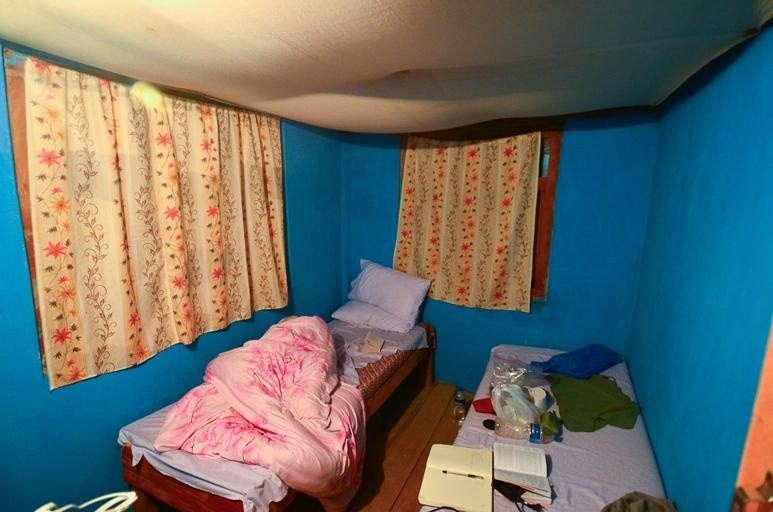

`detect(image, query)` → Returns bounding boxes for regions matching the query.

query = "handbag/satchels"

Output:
[544,373,640,432]
[531,344,623,380]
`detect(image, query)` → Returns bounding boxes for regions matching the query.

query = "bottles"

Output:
[494,417,563,443]
[452,384,465,427]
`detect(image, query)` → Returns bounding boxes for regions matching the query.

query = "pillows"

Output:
[331,300,418,334]
[346,259,433,323]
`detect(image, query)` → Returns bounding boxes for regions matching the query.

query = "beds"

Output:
[117,323,437,512]
[421,343,671,512]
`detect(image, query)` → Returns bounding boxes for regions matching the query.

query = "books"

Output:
[363,331,386,352]
[418,442,553,512]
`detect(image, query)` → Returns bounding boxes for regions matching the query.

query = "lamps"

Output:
[129,81,161,107]
